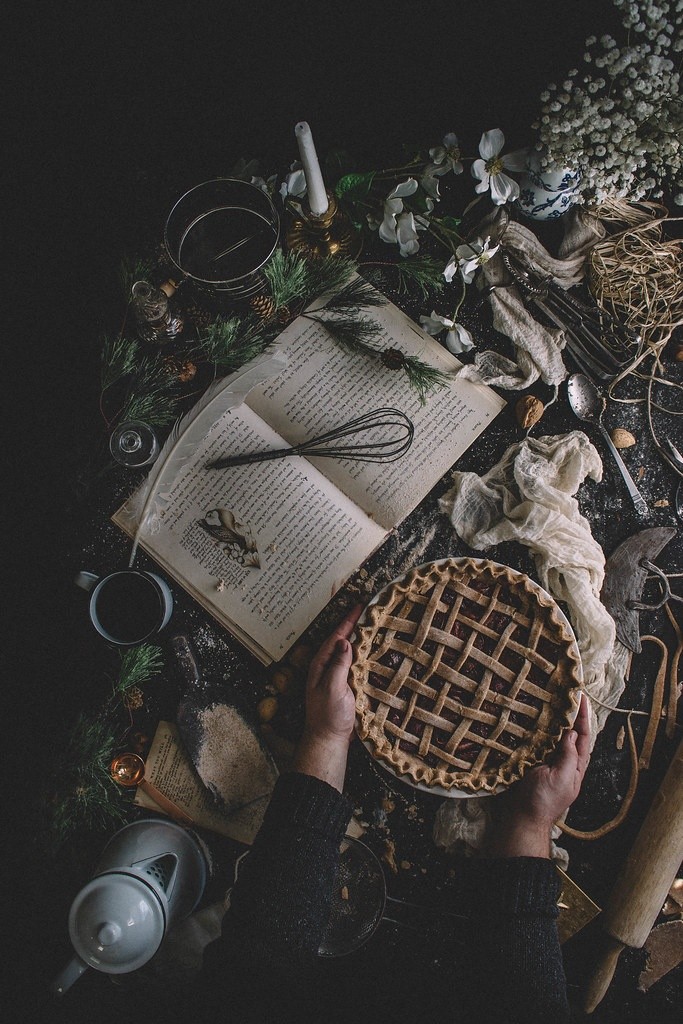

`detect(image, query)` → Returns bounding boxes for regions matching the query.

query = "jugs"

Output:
[43,819,206,995]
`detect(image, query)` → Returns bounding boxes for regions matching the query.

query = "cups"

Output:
[163,179,281,303]
[72,569,173,645]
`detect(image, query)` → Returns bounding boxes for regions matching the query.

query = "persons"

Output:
[186,603,593,1024]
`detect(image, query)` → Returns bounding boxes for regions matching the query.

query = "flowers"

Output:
[229,129,529,352]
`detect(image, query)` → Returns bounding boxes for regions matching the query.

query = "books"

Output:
[108,268,509,666]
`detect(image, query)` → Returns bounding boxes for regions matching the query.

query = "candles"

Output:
[295,121,329,214]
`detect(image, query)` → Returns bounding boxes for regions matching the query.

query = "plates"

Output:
[347,557,584,799]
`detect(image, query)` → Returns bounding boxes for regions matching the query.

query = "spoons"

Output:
[567,374,648,515]
[111,753,193,829]
